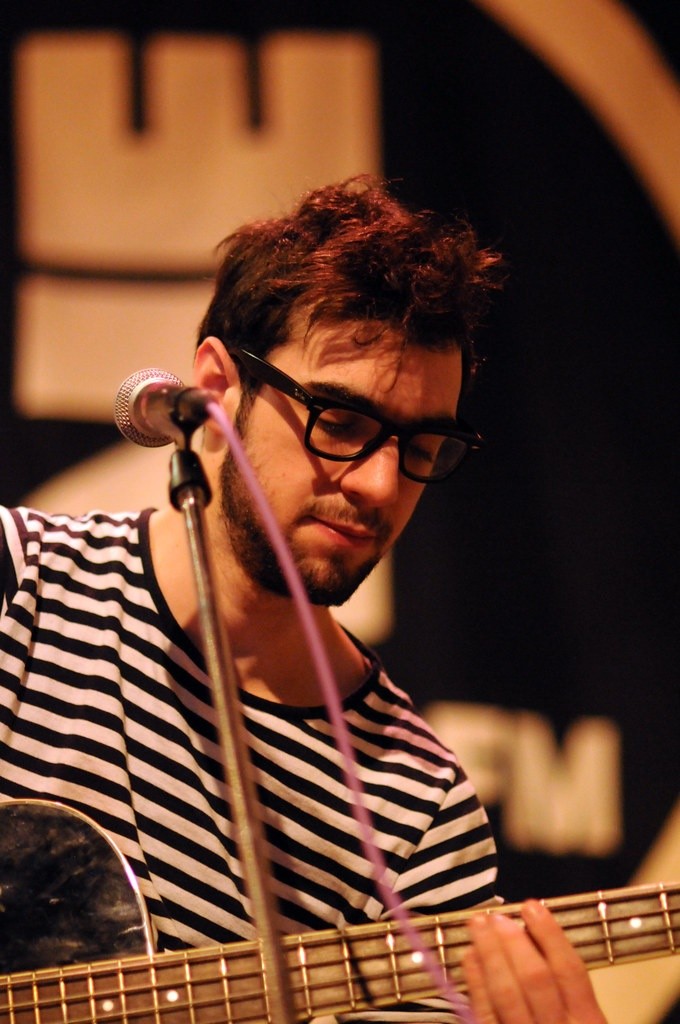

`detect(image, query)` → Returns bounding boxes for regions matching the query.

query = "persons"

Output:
[0,175,606,1023]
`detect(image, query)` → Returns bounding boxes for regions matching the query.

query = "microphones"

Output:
[114,368,220,448]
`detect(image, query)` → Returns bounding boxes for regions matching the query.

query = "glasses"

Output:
[228,345,489,485]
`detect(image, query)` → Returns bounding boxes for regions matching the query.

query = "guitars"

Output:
[0,793,679,1024]
